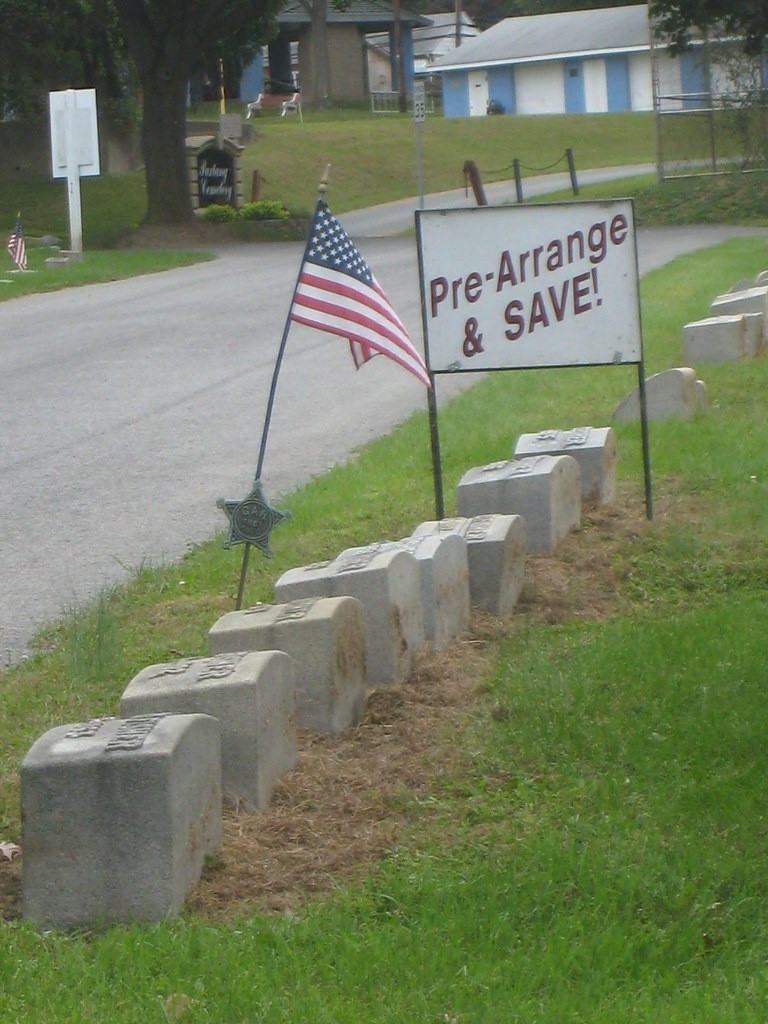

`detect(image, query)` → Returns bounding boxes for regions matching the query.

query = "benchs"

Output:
[246,92,302,120]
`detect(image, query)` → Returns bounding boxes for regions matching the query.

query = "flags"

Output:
[7,221,27,273]
[288,199,432,389]
